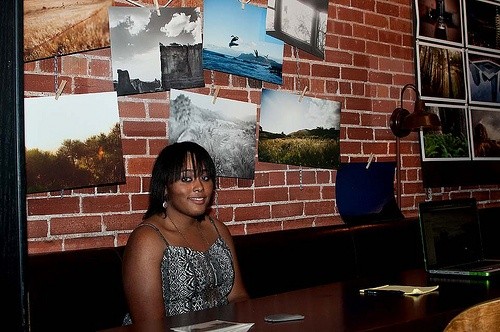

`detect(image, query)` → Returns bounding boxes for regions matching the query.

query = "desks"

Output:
[106,269,500,332]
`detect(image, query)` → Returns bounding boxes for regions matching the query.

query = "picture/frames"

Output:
[412,0,500,162]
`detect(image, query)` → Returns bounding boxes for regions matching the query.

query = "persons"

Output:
[122,141,249,326]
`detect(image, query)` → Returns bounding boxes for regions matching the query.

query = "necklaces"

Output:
[165,210,210,264]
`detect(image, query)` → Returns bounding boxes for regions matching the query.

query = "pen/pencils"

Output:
[364,289,406,295]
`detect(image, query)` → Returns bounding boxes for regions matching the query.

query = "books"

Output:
[359,285,439,294]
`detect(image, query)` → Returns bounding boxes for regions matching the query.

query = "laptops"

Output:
[417,197,499,280]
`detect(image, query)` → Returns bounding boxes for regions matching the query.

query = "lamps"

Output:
[389,83,442,138]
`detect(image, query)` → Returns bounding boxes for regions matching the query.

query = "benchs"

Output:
[27,206,500,332]
